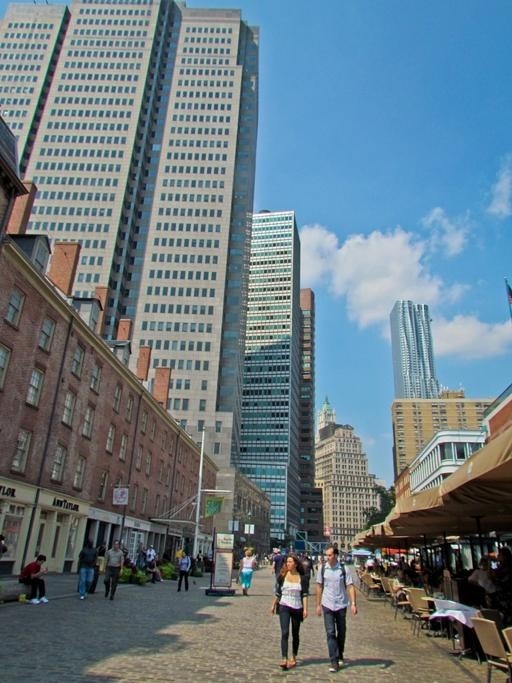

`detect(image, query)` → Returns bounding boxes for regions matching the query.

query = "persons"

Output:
[103,540,124,600]
[139,542,204,591]
[77,538,97,599]
[272,555,308,668]
[236,550,257,595]
[1,534,7,556]
[268,547,314,594]
[315,545,357,672]
[365,548,512,623]
[20,554,48,605]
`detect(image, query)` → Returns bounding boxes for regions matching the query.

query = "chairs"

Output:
[355,568,512,683]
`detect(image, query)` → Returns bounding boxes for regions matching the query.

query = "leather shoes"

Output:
[279,661,296,669]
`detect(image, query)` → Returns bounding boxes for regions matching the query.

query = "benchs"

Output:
[147,573,154,580]
[0,582,32,602]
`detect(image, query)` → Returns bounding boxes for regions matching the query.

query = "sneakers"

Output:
[329,663,339,673]
[80,593,88,600]
[29,599,40,605]
[39,597,48,603]
